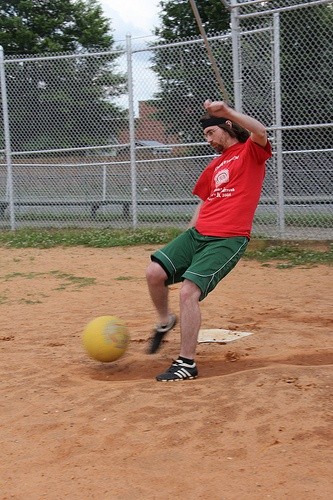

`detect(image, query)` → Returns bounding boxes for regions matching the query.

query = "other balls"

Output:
[82,315,129,364]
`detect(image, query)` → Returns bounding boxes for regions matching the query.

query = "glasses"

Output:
[203,127,219,140]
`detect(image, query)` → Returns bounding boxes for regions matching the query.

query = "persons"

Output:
[143,97,273,381]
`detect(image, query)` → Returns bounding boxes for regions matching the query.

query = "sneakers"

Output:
[149,317,177,354]
[156,361,198,382]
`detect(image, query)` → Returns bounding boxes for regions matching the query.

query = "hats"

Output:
[198,113,228,130]
